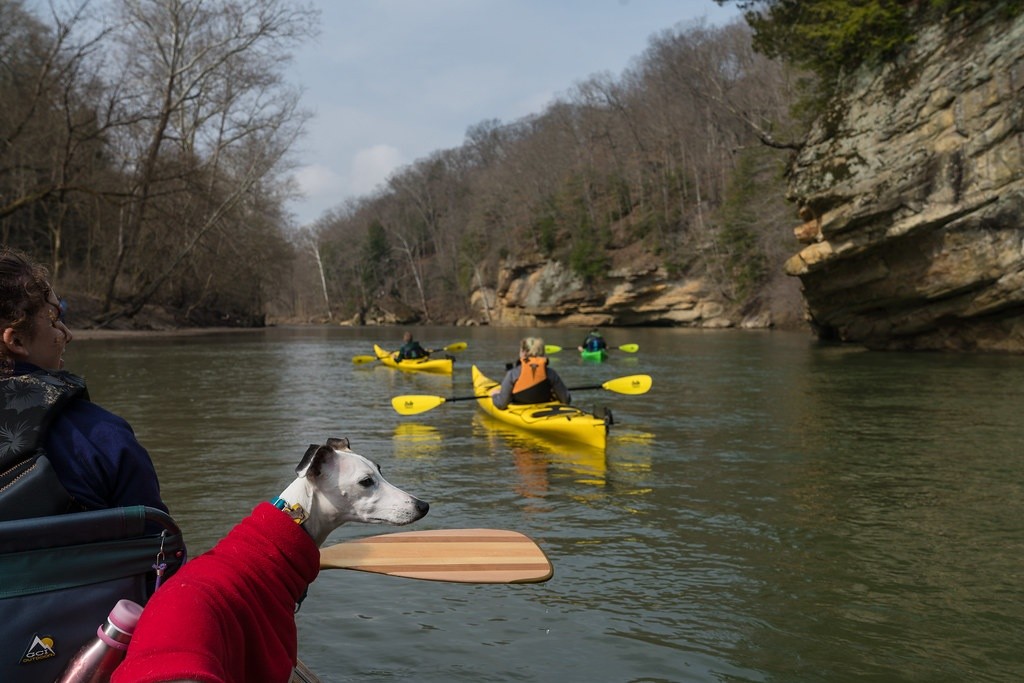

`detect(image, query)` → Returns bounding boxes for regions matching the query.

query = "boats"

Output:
[579,346,608,366]
[372,343,453,375]
[470,363,614,449]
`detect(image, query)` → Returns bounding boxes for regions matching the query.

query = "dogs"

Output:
[110,437,430,683]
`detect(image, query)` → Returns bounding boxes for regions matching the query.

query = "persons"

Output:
[392,331,431,363]
[1,249,172,524]
[490,336,572,410]
[577,328,609,352]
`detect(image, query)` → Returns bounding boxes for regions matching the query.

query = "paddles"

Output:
[391,374,653,417]
[318,526,555,584]
[544,343,639,355]
[351,342,468,367]
